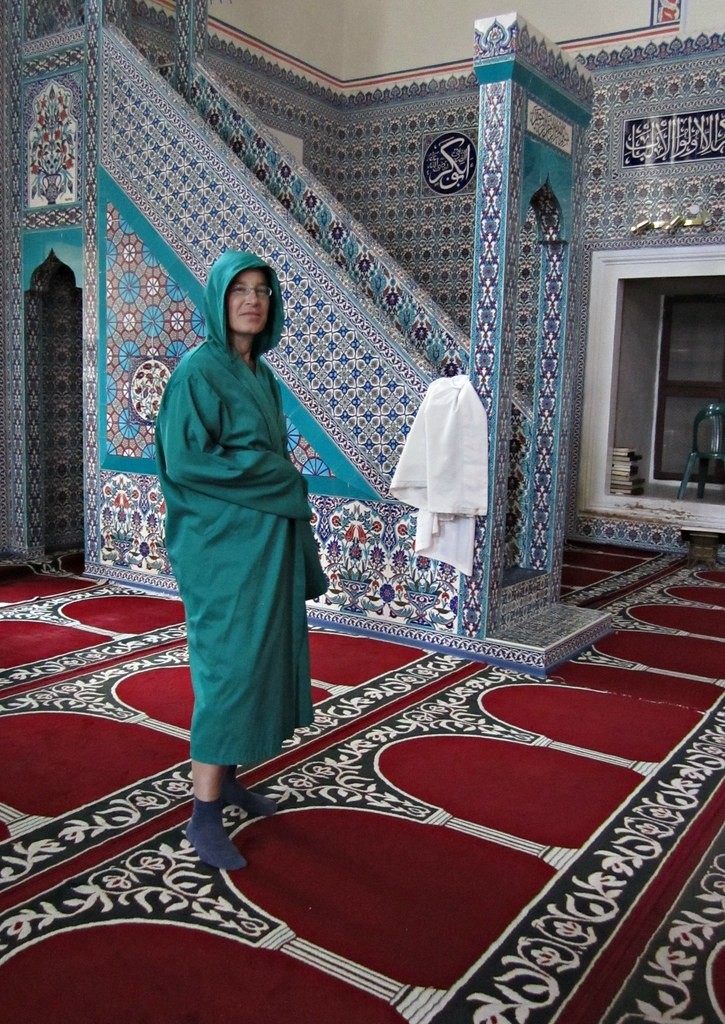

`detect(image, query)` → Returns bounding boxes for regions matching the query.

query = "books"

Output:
[609,446,646,497]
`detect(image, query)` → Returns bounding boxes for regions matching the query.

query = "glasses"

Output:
[230,282,273,299]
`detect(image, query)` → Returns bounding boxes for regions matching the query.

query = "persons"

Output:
[152,246,330,873]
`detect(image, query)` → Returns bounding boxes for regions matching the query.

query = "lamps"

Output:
[630,219,652,238]
[700,207,712,228]
[661,215,683,236]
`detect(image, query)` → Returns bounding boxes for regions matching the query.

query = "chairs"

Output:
[676,402,725,500]
